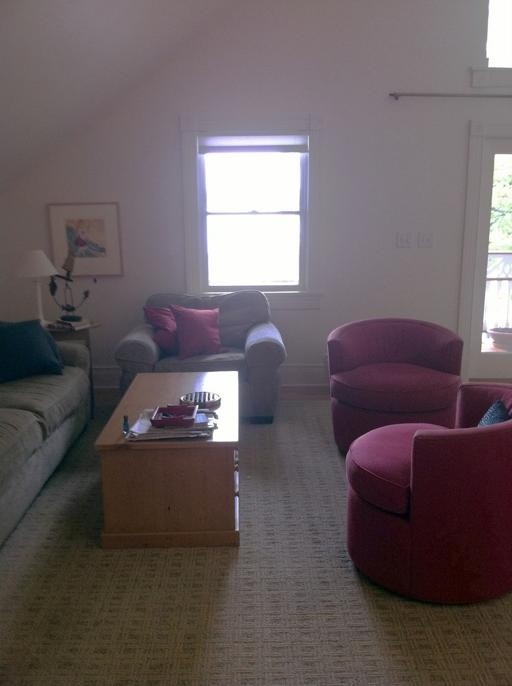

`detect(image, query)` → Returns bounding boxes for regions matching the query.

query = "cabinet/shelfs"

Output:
[95,370,243,549]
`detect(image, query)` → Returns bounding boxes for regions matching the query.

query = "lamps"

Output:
[10,249,60,327]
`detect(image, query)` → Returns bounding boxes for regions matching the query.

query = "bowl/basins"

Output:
[489,327,512,343]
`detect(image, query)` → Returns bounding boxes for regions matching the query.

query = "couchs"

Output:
[114,289,288,424]
[0,340,92,549]
[327,316,464,453]
[346,381,511,605]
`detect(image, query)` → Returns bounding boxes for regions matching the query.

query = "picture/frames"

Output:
[46,201,125,277]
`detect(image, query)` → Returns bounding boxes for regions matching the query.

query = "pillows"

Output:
[144,306,177,354]
[1,319,64,382]
[477,399,511,426]
[170,303,229,360]
[61,351,77,367]
